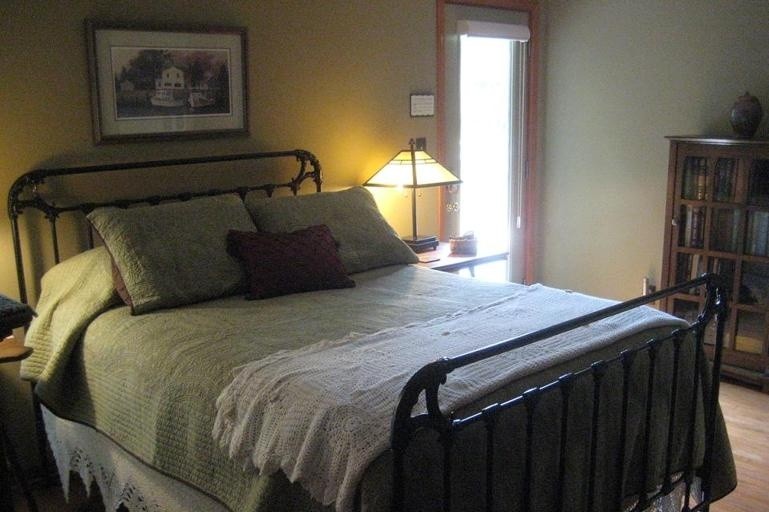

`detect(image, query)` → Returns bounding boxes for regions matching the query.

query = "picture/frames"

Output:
[82,15,249,137]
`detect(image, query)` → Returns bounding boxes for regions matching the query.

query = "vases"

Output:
[730,91,763,139]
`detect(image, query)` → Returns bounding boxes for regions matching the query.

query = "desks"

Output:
[410,239,510,277]
[0,337,38,512]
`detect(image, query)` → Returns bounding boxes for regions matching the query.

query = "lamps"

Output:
[363,139,462,250]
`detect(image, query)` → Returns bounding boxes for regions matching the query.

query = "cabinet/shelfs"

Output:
[659,134,769,394]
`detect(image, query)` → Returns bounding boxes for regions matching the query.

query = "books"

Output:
[674,153,769,310]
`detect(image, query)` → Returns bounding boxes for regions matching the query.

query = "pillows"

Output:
[88,189,259,318]
[246,184,420,273]
[225,221,357,299]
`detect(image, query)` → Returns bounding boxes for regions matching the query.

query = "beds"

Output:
[7,145,736,512]
